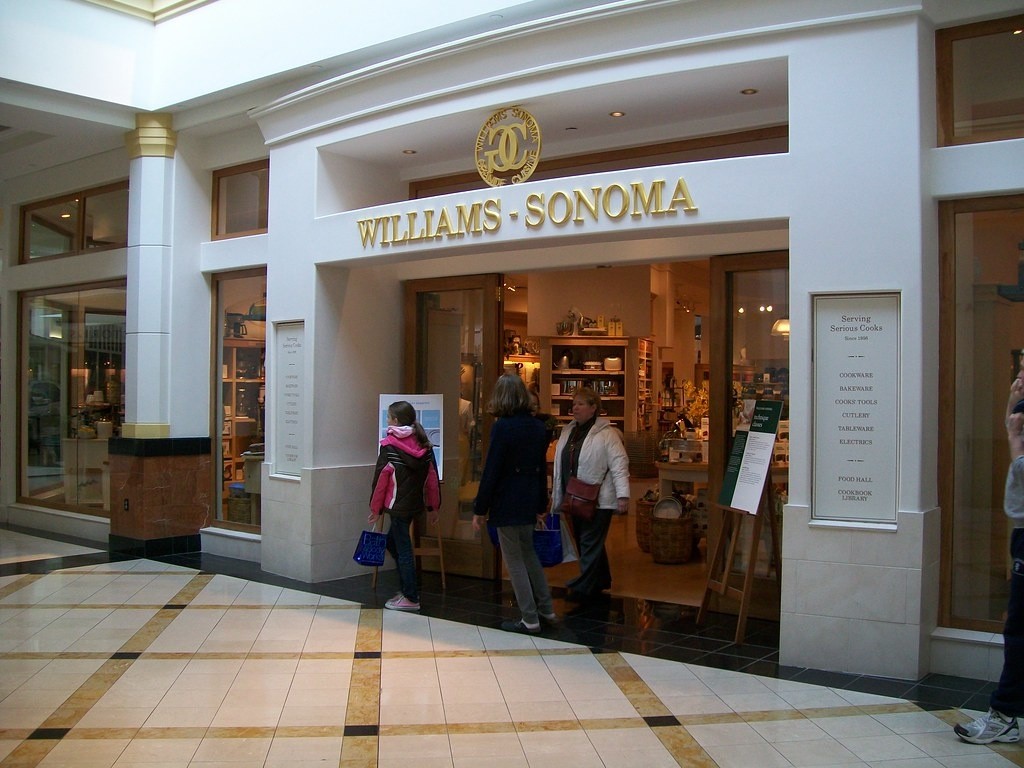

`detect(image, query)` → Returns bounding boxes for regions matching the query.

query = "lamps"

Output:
[770,316,790,337]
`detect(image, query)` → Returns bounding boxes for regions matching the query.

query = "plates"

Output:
[652,496,683,519]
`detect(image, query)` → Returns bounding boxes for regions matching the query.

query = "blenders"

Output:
[225,312,247,339]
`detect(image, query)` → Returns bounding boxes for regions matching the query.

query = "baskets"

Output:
[641,508,693,564]
[634,499,658,553]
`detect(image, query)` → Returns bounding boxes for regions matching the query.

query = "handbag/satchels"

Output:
[533,519,563,567]
[559,478,601,519]
[352,530,388,566]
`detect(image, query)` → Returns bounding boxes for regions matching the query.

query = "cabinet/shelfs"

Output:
[694,363,756,393]
[222,337,266,501]
[539,335,655,464]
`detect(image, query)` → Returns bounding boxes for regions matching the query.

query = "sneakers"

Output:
[536,610,558,624]
[565,576,612,600]
[954,707,1020,743]
[501,617,540,632]
[385,593,420,609]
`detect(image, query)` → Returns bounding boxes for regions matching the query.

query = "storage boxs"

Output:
[551,383,561,396]
[222,364,228,379]
[228,482,251,498]
[615,322,623,336]
[224,405,231,417]
[222,440,230,457]
[775,442,786,463]
[702,442,708,463]
[583,360,602,372]
[552,404,561,416]
[700,417,710,441]
[235,469,243,480]
[785,443,789,463]
[598,315,605,328]
[223,460,232,481]
[223,421,232,436]
[776,420,789,441]
[608,322,615,336]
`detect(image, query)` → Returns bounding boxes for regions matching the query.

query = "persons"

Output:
[369,401,442,611]
[954,348,1023,745]
[550,386,630,598]
[473,373,557,636]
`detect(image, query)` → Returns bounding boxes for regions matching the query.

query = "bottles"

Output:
[237,389,248,415]
[97,417,112,438]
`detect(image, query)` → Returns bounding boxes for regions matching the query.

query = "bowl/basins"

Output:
[86,395,96,402]
[77,429,95,439]
[94,391,104,401]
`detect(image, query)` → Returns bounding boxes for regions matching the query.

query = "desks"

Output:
[653,460,789,501]
[60,438,110,511]
[243,456,265,525]
